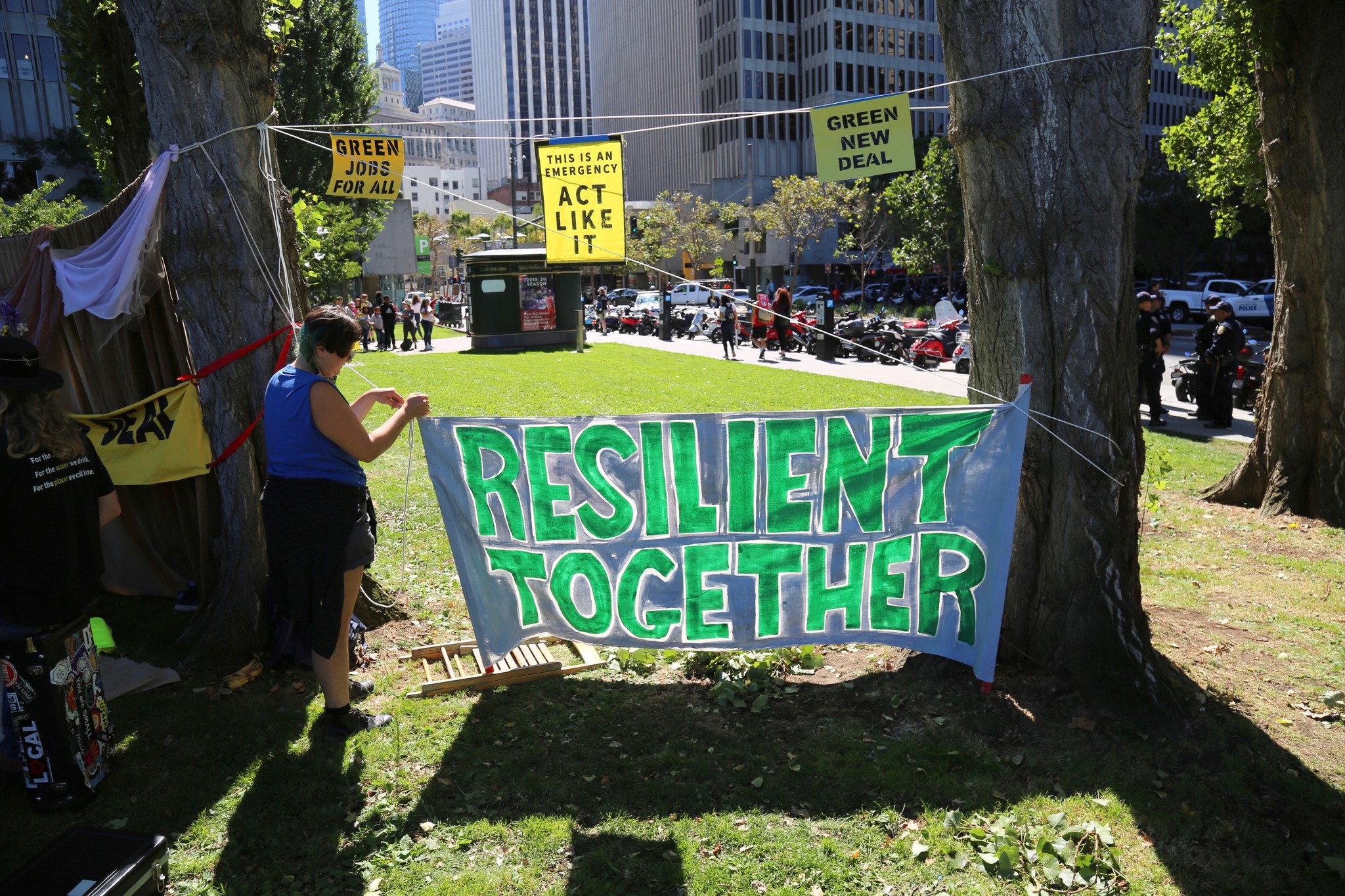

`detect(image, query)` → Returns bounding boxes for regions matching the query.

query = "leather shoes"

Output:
[1149,405,1225,430]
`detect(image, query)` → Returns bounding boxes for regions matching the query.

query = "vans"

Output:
[670,279,736,309]
[1184,271,1226,287]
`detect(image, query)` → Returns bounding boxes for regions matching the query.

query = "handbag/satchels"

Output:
[593,294,603,314]
[778,312,790,325]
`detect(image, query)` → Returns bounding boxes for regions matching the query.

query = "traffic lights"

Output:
[877,250,882,262]
[453,278,457,284]
[733,254,737,265]
[864,247,868,258]
[456,248,465,264]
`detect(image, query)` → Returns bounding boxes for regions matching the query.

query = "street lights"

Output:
[507,130,559,248]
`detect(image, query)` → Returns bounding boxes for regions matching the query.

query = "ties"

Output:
[1209,301,1232,312]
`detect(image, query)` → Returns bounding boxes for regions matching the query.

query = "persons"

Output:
[750,290,770,359]
[666,282,672,291]
[650,285,656,291]
[334,290,460,351]
[674,282,679,288]
[714,294,739,358]
[521,274,556,330]
[771,287,792,359]
[714,276,967,307]
[261,305,430,738]
[596,287,611,336]
[1,335,122,649]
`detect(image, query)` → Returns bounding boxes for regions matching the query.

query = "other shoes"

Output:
[425,345,436,349]
[732,350,736,357]
[363,338,396,353]
[602,331,607,336]
[759,348,766,358]
[550,326,552,329]
[544,326,546,330]
[778,352,785,358]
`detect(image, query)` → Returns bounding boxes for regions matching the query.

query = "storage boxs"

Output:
[842,319,865,333]
[902,317,929,337]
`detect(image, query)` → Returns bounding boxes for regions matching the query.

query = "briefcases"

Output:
[0,610,118,814]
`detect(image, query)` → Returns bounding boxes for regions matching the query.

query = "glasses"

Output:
[347,349,357,364]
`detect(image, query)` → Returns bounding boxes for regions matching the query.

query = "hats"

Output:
[1202,294,1221,304]
[548,290,552,292]
[597,287,604,292]
[349,301,356,308]
[402,299,412,307]
[1136,291,1157,303]
[0,335,64,391]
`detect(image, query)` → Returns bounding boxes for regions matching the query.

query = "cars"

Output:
[1226,279,1276,331]
[597,288,639,307]
[634,290,660,313]
[1133,277,1176,292]
[734,289,750,307]
[792,284,829,308]
[405,292,425,299]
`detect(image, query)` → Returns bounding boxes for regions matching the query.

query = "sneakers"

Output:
[324,707,393,741]
[348,677,375,703]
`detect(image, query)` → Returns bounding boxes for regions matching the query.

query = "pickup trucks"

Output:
[1158,278,1265,327]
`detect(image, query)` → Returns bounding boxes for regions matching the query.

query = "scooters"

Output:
[1172,328,1272,411]
[908,300,968,372]
[953,331,970,374]
[587,283,967,367]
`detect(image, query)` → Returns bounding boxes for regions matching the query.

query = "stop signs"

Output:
[825,263,831,274]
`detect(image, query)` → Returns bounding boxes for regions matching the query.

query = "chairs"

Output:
[396,618,611,701]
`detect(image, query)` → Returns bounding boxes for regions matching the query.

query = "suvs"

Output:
[839,283,891,306]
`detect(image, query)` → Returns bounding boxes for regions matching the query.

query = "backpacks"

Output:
[400,338,413,351]
[721,303,736,321]
[361,301,374,318]
[754,301,772,324]
[346,612,367,675]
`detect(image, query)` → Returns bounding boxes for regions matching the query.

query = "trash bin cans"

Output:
[438,301,463,328]
